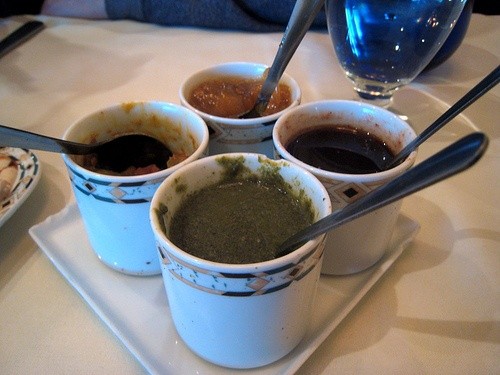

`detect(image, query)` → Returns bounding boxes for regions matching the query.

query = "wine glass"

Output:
[323,0,465,125]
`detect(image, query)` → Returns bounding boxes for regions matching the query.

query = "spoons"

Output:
[0,124,172,174]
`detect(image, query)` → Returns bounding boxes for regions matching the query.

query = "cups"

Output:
[178,61,300,159]
[60,99,210,276]
[150,151,332,370]
[272,98,417,277]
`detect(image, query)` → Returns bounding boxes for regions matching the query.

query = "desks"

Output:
[0,12,500,375]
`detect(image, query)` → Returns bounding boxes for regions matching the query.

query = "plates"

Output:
[0,145,41,228]
[27,201,421,375]
